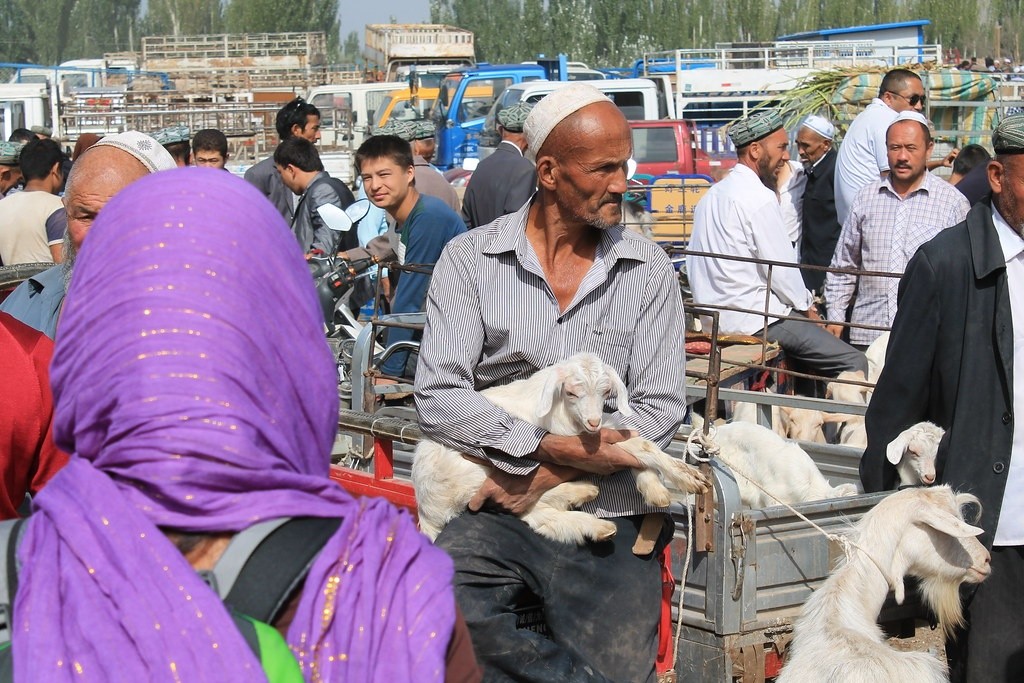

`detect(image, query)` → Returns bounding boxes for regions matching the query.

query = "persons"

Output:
[859,116,1024,683]
[775,116,842,299]
[244,99,324,228]
[834,70,960,229]
[273,135,359,260]
[949,143,990,185]
[828,111,971,354]
[463,101,537,231]
[0,125,480,683]
[415,85,687,683]
[686,108,868,403]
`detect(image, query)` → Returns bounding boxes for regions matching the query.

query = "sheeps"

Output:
[826,369,872,450]
[412,352,712,542]
[688,410,856,510]
[734,389,851,445]
[887,421,945,485]
[775,486,991,682]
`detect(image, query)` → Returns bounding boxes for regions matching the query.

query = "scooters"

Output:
[291,198,901,683]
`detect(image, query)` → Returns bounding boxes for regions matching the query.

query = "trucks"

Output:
[0,24,1024,314]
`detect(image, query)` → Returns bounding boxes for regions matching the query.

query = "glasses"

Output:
[889,91,926,106]
[288,97,304,121]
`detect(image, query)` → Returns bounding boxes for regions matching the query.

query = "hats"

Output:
[154,126,191,145]
[886,110,930,131]
[728,110,783,147]
[83,130,178,173]
[0,141,24,164]
[994,60,1000,65]
[498,103,533,132]
[31,126,52,137]
[523,83,616,161]
[992,115,1024,154]
[803,114,834,140]
[414,120,436,140]
[1004,59,1011,64]
[372,122,414,142]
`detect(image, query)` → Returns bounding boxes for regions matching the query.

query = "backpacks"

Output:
[1,516,345,683]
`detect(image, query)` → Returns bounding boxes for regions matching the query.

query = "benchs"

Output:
[682,340,779,424]
